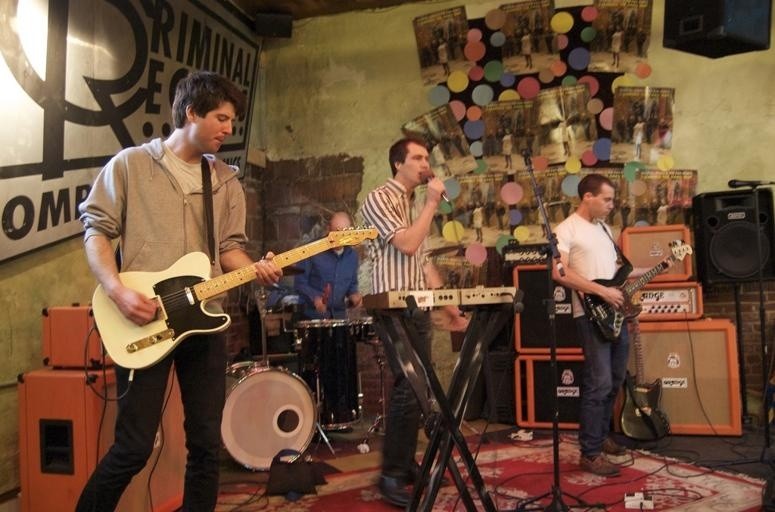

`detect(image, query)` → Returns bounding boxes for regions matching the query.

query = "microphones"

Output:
[420,166,453,208]
[727,177,772,189]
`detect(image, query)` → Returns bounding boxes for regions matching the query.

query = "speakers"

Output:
[691,188,775,286]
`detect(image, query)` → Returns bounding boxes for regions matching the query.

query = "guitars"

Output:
[584,239,693,341]
[91,222,377,370]
[621,316,670,441]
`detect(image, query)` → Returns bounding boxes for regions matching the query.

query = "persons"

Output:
[353,137,464,508]
[295,212,362,423]
[551,174,631,477]
[75,70,285,512]
[424,25,690,242]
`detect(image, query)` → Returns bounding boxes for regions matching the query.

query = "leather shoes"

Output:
[380,486,413,507]
[407,465,447,488]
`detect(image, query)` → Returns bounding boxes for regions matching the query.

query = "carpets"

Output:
[214,432,766,511]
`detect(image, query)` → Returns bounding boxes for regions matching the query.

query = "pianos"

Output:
[363,283,516,309]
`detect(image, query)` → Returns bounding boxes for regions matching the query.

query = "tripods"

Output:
[513,145,601,512]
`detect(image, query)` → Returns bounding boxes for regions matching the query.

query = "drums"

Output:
[221,360,318,472]
[292,318,362,428]
[353,316,384,345]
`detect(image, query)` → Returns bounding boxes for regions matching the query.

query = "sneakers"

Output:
[602,439,626,455]
[579,452,621,477]
